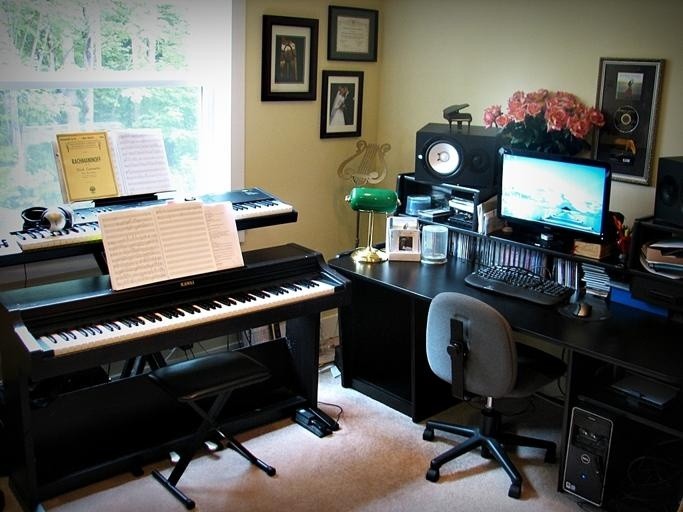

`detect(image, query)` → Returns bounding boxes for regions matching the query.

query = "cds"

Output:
[613,105,639,134]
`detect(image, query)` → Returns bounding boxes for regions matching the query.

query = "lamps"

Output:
[341,187,404,266]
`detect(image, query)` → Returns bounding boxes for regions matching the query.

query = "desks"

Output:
[327,170,683,511]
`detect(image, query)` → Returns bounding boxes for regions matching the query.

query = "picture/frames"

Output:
[258,12,318,102]
[321,69,363,139]
[389,229,419,253]
[590,55,667,187]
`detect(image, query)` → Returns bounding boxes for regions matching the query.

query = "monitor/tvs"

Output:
[497,146,612,248]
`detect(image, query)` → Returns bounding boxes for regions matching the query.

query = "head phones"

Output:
[21,206,74,231]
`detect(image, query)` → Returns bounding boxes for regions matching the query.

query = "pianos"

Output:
[0,186,298,268]
[0,244,352,512]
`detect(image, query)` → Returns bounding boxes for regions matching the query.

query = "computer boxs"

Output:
[562,405,621,508]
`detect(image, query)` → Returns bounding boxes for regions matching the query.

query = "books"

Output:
[447,196,628,298]
[52,126,246,293]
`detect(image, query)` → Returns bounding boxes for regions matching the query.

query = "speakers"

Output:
[415,123,505,188]
[653,156,683,229]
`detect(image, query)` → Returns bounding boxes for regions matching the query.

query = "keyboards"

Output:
[464,265,573,305]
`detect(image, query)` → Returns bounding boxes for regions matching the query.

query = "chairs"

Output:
[422,287,559,499]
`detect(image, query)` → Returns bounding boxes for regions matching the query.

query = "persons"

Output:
[330,91,345,126]
[624,79,635,95]
[337,84,354,126]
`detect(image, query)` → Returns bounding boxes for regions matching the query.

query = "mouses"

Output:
[573,302,587,316]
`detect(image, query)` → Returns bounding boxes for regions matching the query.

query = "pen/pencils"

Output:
[612,217,633,237]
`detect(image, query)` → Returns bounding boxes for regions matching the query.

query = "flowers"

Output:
[480,86,607,158]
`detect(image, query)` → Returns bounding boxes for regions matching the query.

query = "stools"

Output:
[146,348,276,511]
